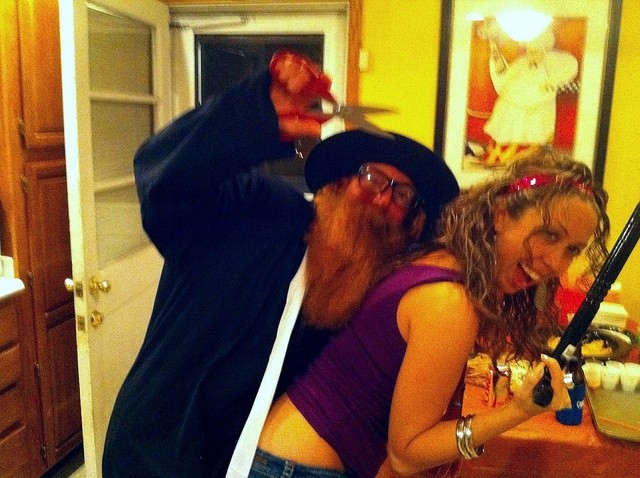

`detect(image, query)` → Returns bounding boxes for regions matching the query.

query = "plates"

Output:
[583,324,635,361]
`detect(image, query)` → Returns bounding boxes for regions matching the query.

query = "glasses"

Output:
[358,166,415,208]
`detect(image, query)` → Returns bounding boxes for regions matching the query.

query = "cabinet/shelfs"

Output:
[1,0,83,477]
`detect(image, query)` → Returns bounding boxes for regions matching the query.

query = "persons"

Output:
[103,51,461,478]
[248,144,610,478]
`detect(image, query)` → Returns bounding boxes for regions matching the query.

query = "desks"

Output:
[444,297,640,478]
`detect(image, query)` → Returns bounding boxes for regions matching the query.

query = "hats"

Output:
[304,130,460,209]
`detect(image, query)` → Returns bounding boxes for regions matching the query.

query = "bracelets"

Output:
[456,413,485,460]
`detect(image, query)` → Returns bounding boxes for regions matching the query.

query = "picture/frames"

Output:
[433,0,624,191]
